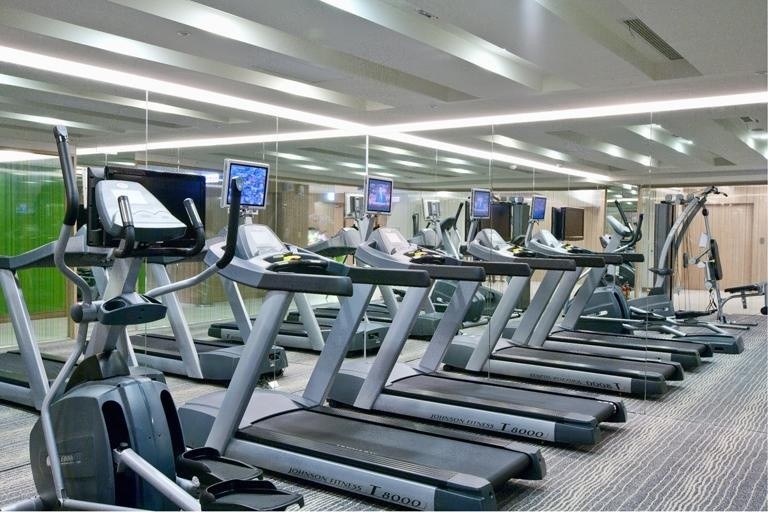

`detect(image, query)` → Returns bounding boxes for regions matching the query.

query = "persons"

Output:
[376,186,387,204]
[475,197,486,211]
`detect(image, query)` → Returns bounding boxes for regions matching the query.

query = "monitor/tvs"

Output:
[551,206,564,241]
[471,189,492,220]
[424,199,441,220]
[561,207,585,240]
[87,166,102,246]
[531,195,547,219]
[104,168,204,248]
[343,192,363,219]
[366,176,393,215]
[218,156,270,210]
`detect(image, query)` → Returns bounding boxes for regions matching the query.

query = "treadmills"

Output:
[327,174,630,454]
[178,156,547,510]
[524,194,713,372]
[81,199,290,388]
[0,166,169,413]
[439,186,687,399]
[208,194,507,353]
